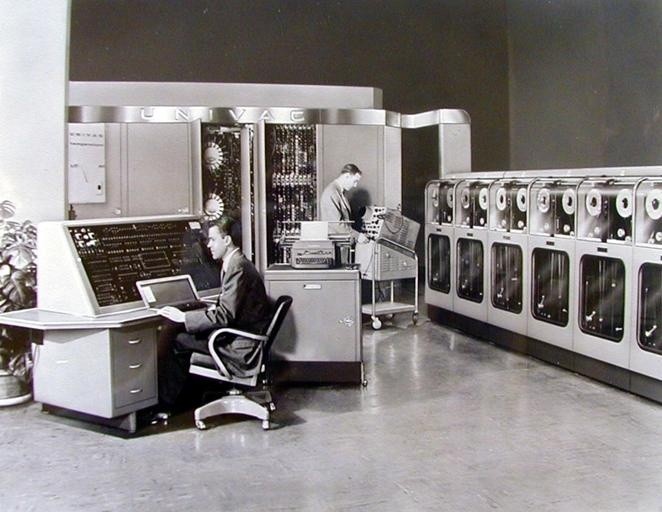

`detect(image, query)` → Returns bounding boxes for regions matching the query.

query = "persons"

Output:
[318,164,371,245]
[139,215,268,426]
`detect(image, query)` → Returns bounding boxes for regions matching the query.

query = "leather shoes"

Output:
[140,412,171,427]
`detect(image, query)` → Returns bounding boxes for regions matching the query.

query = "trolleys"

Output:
[354,234,419,332]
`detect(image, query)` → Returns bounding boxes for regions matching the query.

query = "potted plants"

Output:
[0,212,39,406]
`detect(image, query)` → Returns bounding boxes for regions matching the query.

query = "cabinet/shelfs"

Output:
[10,269,167,421]
[266,257,368,390]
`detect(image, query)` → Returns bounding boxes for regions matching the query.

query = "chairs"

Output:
[187,293,294,433]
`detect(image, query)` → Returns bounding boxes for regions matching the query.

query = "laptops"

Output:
[135,274,207,313]
